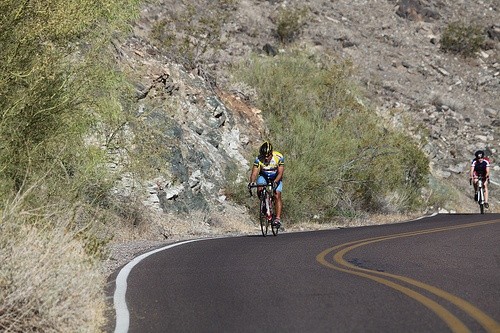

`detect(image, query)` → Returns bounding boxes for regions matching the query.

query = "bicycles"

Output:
[470,176,491,215]
[247,181,281,237]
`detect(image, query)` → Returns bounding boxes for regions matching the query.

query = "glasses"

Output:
[264,155,271,158]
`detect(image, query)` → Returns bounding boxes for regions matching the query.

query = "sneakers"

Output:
[474,194,478,201]
[484,203,489,208]
[271,217,281,229]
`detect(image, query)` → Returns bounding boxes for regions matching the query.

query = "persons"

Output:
[470,151,491,209]
[248,141,285,228]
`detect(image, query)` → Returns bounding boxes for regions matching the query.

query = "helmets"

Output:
[259,141,273,154]
[475,151,484,158]
